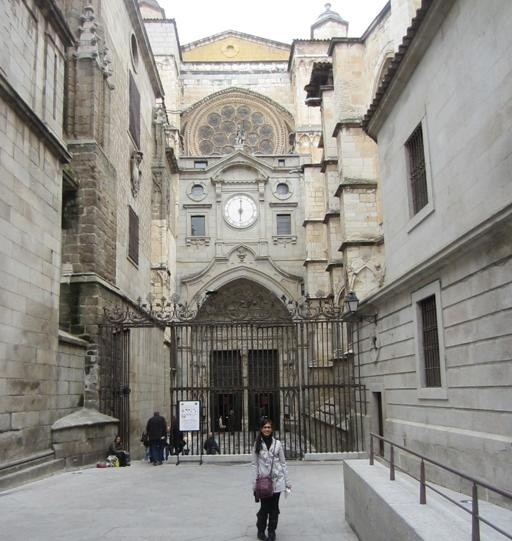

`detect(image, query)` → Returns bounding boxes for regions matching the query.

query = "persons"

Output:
[228,410,236,435]
[107,435,130,467]
[250,415,292,541]
[141,411,192,466]
[203,432,221,455]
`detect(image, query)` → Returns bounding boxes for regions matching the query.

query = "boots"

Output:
[256,513,278,540]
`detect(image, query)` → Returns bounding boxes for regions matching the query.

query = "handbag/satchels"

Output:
[254,476,274,498]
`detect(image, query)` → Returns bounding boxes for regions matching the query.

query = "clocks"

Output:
[222,192,260,230]
